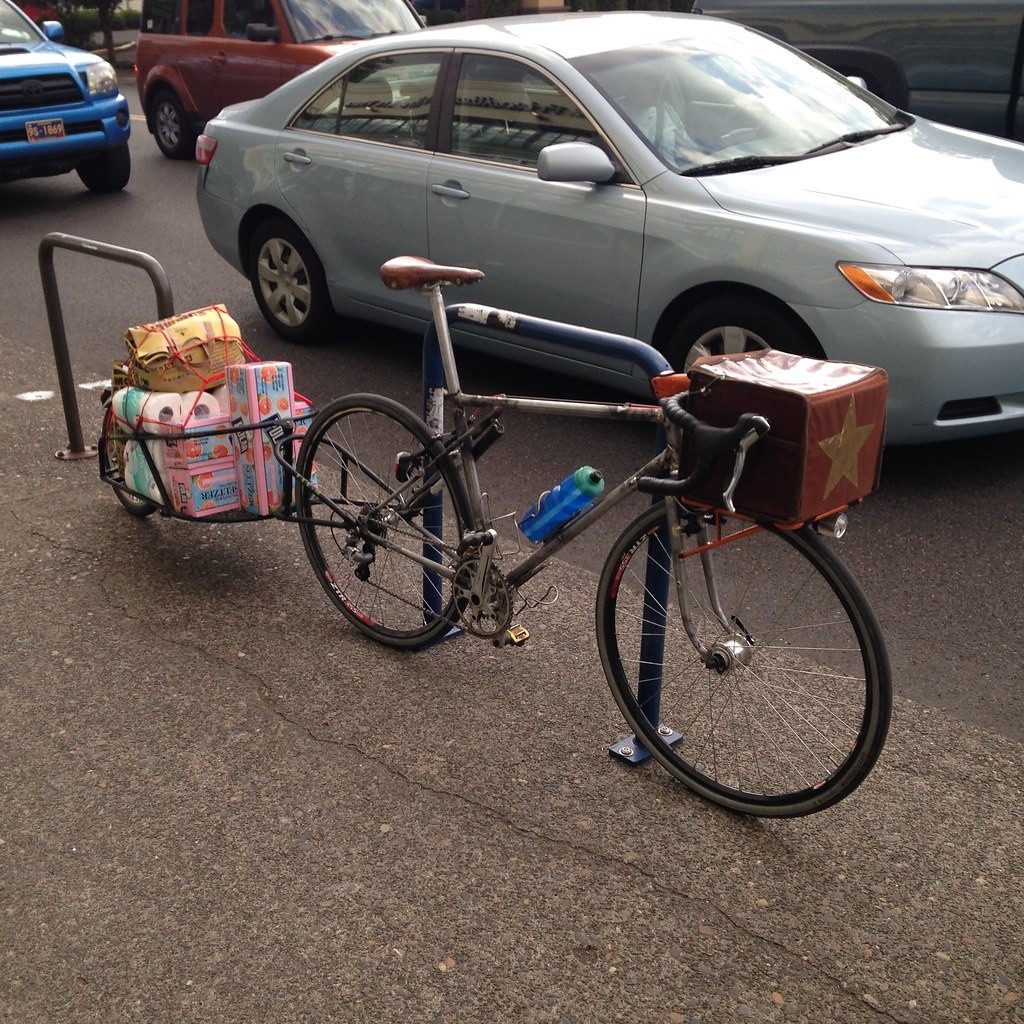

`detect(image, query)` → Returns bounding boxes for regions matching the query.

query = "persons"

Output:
[592,73,708,184]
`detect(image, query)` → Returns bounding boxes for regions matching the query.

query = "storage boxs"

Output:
[161,361,318,520]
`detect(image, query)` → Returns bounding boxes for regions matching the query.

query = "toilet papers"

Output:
[180,381,230,423]
[122,438,173,507]
[112,385,184,436]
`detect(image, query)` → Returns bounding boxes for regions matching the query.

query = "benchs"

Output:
[323,75,436,151]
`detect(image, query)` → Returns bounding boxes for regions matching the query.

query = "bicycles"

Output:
[272,256,891,819]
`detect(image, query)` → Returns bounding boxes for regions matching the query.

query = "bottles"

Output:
[519,467,604,544]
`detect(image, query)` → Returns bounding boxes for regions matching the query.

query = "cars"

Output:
[134,1,429,158]
[0,0,130,193]
[194,12,1024,445]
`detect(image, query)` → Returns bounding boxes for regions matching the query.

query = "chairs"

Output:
[456,80,537,166]
